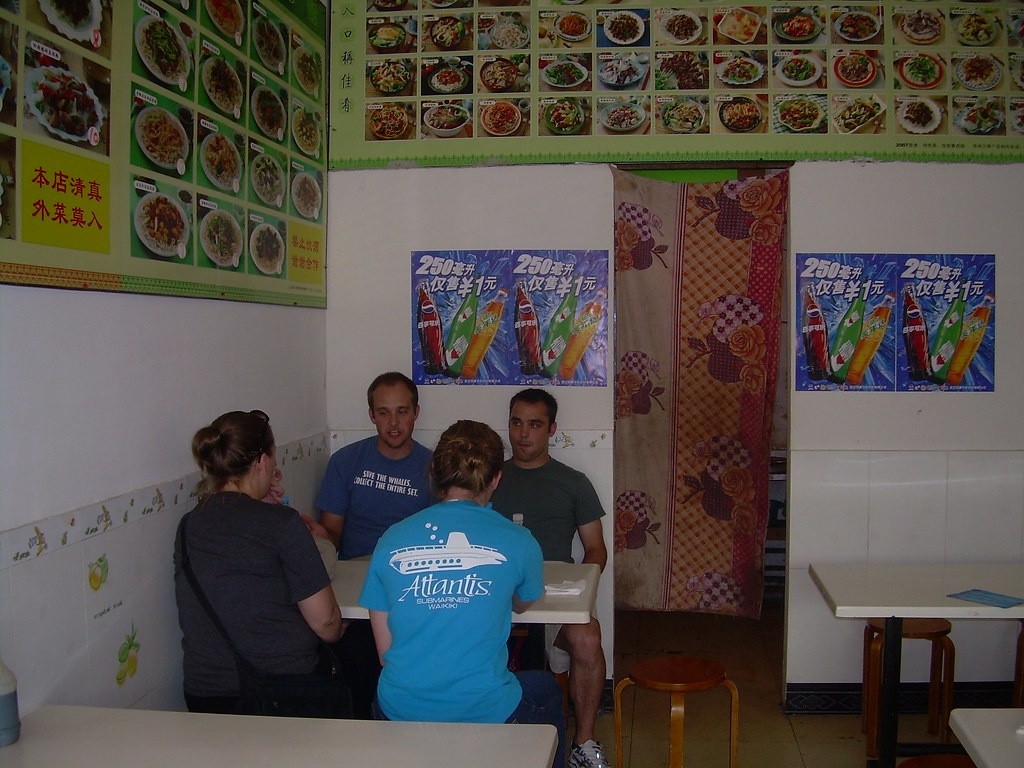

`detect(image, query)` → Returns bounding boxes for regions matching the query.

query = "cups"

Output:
[447,57,460,68]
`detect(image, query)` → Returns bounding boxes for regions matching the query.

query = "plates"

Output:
[553,11,593,42]
[24,66,105,142]
[716,7,765,132]
[774,11,1024,135]
[478,11,530,49]
[659,10,702,45]
[481,101,521,135]
[372,0,458,7]
[542,61,588,135]
[40,0,103,42]
[427,66,468,93]
[370,104,409,139]
[0,55,12,112]
[598,11,646,131]
[135,0,321,275]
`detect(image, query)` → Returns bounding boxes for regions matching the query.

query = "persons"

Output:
[172,409,375,720]
[486,389,611,768]
[300,372,434,699]
[357,417,566,768]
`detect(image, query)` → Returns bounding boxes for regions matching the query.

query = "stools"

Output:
[613,653,739,768]
[862,618,955,757]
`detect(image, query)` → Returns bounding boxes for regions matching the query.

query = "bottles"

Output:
[947,294,995,384]
[558,291,607,380]
[460,287,511,379]
[931,280,972,382]
[802,283,830,381]
[830,282,871,382]
[445,273,483,378]
[846,293,895,385]
[904,285,931,381]
[418,278,446,375]
[542,276,583,377]
[513,513,523,526]
[515,277,545,375]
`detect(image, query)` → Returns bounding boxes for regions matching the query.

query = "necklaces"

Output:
[446,495,473,498]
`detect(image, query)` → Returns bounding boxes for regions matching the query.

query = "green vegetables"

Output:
[857,56,870,67]
[546,63,583,85]
[369,27,405,47]
[906,57,938,83]
[509,54,526,65]
[729,64,759,82]
[654,68,680,90]
[372,67,407,90]
[445,106,468,122]
[455,22,464,38]
[783,57,813,80]
[660,96,703,129]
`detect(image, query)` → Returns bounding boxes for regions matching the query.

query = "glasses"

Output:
[251,409,269,464]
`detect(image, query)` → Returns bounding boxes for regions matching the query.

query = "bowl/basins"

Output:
[659,99,706,134]
[369,23,406,54]
[480,58,518,93]
[430,16,465,51]
[370,62,411,96]
[424,104,470,137]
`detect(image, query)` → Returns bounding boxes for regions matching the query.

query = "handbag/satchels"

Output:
[234,652,347,719]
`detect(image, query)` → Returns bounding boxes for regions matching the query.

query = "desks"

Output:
[948,708,1024,768]
[810,563,1024,708]
[0,703,558,768]
[325,560,601,673]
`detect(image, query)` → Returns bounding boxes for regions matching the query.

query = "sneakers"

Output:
[567,736,611,768]
